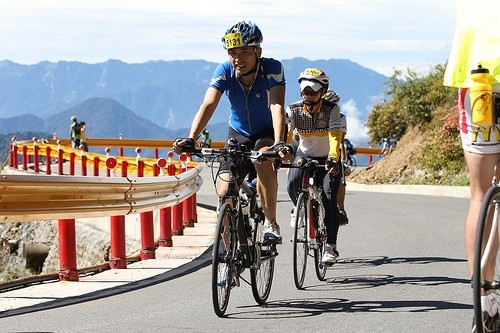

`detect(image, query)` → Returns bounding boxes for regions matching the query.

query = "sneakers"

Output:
[471,308,500,333]
[290,204,305,229]
[262,220,283,245]
[321,244,338,264]
[216,274,240,287]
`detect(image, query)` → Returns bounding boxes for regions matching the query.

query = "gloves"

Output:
[325,158,339,176]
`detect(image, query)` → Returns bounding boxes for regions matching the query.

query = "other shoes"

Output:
[339,209,348,226]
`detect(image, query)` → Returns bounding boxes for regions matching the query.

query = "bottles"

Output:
[239,195,249,220]
[469,64,493,124]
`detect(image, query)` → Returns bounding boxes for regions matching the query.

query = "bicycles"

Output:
[474,167,500,333]
[281,159,339,289]
[181,138,290,316]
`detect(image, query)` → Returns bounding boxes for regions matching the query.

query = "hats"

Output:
[300,79,324,91]
[71,116,76,120]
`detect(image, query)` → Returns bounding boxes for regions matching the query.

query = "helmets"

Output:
[222,20,263,50]
[320,89,340,105]
[298,68,329,88]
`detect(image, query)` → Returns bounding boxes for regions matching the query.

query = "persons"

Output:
[341,137,355,163]
[307,87,351,224]
[196,127,212,163]
[69,116,81,149]
[78,120,89,152]
[171,19,295,264]
[441,0,500,333]
[380,137,397,155]
[273,67,344,264]
